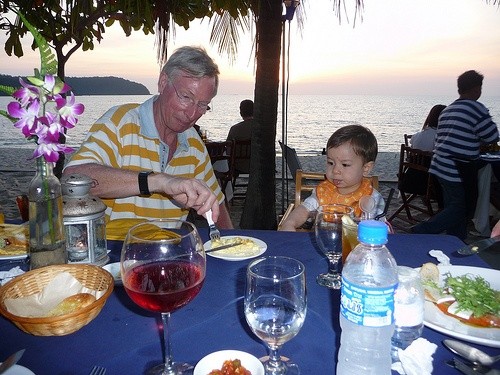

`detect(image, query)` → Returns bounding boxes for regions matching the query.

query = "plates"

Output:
[413,265,500,349]
[192,350,265,375]
[101,259,141,286]
[481,155,500,158]
[203,235,268,261]
[0,223,29,260]
[0,362,36,375]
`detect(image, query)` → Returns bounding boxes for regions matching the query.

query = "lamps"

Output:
[63,173,109,267]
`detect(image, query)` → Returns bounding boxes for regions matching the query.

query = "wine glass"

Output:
[120,219,207,375]
[315,204,355,290]
[243,255,308,375]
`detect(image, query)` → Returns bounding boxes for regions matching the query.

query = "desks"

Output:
[428,151,500,237]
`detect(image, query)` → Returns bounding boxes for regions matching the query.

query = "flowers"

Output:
[0,3,85,250]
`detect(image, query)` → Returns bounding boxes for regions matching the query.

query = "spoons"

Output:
[442,339,500,366]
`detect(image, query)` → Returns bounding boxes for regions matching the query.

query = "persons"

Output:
[411,70,500,239]
[279,124,393,234]
[410,104,447,152]
[225,99,254,169]
[60,47,234,229]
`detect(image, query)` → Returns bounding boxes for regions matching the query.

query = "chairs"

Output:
[385,135,434,236]
[208,137,251,196]
[279,141,312,199]
[296,169,378,232]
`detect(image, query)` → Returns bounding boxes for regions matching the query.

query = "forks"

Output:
[453,357,486,375]
[88,366,107,375]
[203,203,220,242]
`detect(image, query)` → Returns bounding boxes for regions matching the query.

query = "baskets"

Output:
[0,264,115,337]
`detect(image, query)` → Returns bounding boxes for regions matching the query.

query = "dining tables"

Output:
[0,228,500,375]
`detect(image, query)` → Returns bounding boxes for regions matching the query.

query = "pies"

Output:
[0,223,32,255]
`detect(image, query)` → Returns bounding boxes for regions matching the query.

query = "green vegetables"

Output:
[424,271,500,318]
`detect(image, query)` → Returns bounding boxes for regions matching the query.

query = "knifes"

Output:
[0,349,26,374]
[204,243,244,253]
[457,235,500,255]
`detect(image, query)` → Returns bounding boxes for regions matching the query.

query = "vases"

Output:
[30,156,67,268]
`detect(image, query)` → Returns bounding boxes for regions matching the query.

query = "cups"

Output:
[392,265,424,364]
[341,216,386,267]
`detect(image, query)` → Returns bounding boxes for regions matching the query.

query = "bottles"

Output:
[335,219,398,375]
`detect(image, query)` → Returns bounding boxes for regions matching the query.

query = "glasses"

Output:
[167,74,212,115]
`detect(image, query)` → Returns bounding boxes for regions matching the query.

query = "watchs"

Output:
[137,171,152,198]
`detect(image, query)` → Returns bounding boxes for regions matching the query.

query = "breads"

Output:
[49,293,96,317]
